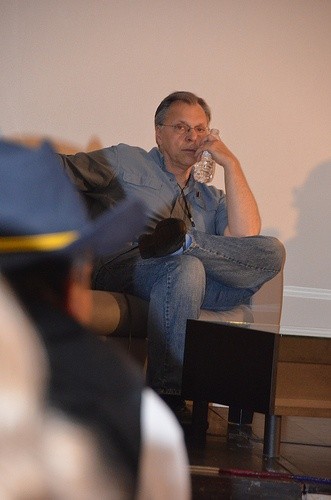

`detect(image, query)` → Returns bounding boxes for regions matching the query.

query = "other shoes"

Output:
[148,218,187,259]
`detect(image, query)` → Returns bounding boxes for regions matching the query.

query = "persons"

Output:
[49,92,289,428]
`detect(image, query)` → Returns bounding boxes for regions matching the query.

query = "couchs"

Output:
[69,263,284,383]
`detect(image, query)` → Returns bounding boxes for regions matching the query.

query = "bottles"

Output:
[193,128,220,183]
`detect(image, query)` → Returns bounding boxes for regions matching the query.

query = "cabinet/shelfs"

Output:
[182,317,331,462]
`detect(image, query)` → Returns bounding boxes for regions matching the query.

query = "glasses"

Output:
[160,124,209,134]
